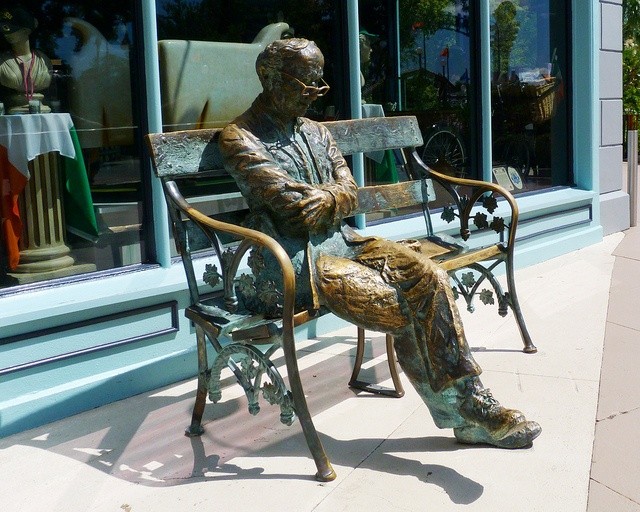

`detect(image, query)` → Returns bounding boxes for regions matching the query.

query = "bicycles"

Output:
[420,81,531,188]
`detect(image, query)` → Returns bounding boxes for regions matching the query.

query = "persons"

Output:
[359,25,382,64]
[217,37,541,449]
[0,8,53,91]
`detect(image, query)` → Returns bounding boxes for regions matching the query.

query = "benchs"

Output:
[145,115,537,481]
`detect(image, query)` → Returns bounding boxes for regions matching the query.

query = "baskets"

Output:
[496,78,558,122]
